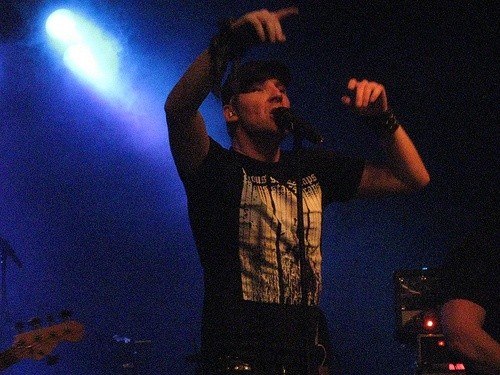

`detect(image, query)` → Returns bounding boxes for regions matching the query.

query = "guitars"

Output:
[0,306,85,375]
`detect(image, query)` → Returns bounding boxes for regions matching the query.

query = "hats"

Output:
[218,46,291,105]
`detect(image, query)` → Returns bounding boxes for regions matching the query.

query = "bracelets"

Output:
[217,14,237,38]
[373,110,400,138]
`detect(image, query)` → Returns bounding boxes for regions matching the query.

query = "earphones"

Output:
[229,111,233,116]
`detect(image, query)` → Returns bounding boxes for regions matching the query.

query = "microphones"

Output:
[273,107,322,144]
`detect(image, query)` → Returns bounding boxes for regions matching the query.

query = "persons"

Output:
[164,8,430,375]
[437,230,499,375]
